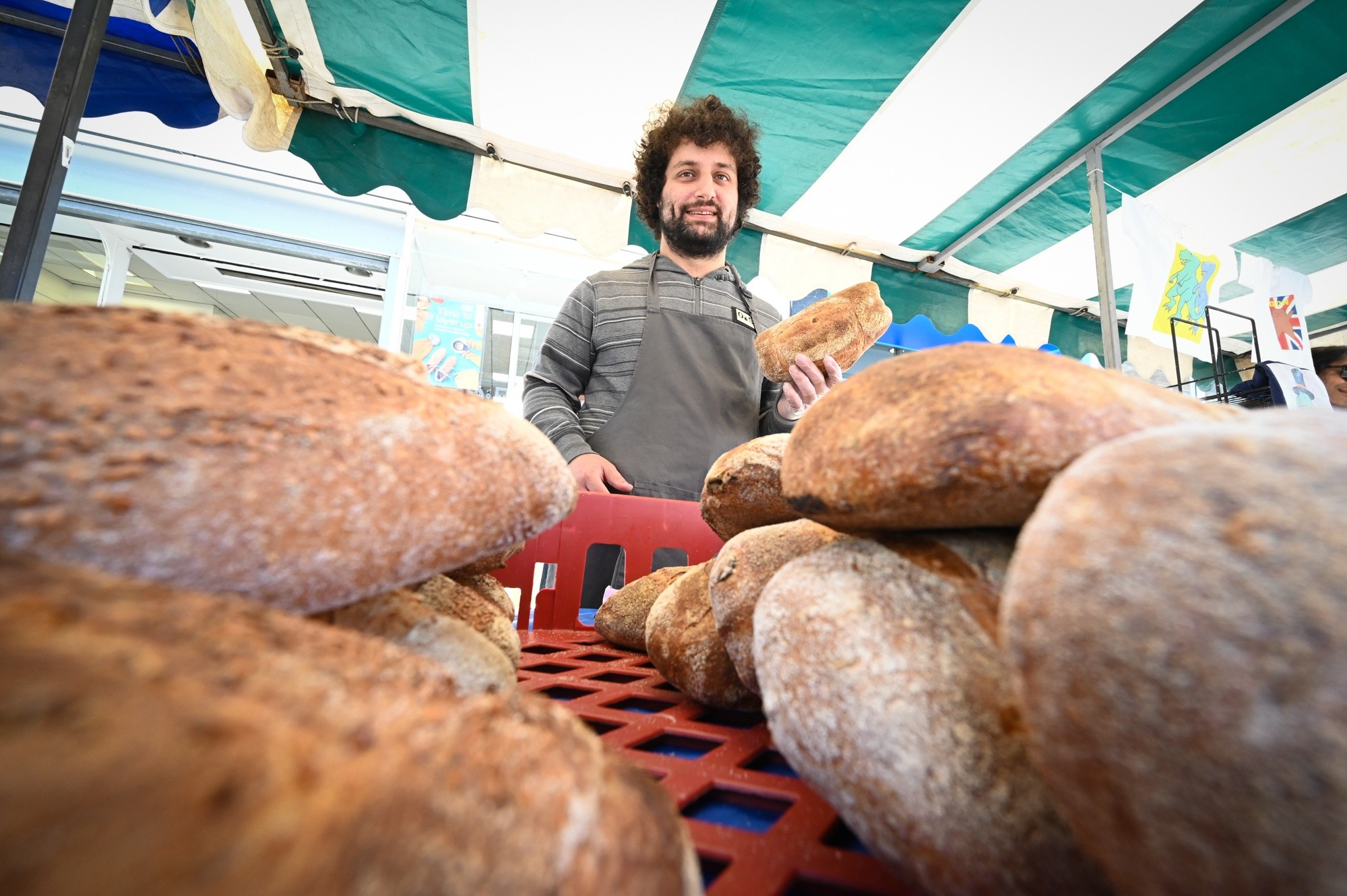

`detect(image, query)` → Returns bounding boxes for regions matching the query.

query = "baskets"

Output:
[489,490,896,896]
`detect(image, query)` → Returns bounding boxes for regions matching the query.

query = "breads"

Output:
[755,281,892,383]
[1,301,708,895]
[592,339,1347,896]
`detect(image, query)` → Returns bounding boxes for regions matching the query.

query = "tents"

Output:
[0,0,1346,414]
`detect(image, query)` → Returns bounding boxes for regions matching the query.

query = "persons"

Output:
[524,95,845,612]
[1310,345,1347,409]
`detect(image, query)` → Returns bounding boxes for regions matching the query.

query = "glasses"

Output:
[1325,364,1347,379]
